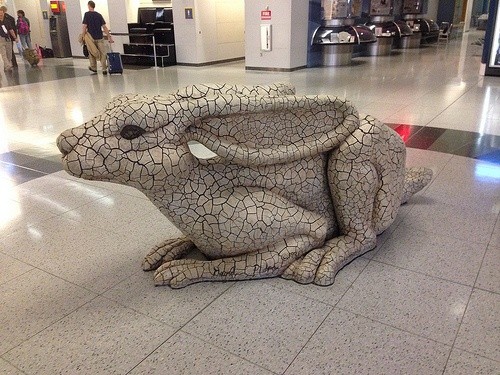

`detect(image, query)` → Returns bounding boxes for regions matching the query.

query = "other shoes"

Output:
[103,71,107,75]
[89,66,97,72]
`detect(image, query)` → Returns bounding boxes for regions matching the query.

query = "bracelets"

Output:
[14,38,17,39]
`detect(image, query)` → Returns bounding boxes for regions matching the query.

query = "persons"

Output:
[16,10,33,49]
[1,6,18,68]
[82,0,113,75]
[0,8,19,73]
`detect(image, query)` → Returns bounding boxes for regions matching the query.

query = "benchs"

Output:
[438,22,466,42]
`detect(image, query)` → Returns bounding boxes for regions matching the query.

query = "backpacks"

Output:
[17,17,29,35]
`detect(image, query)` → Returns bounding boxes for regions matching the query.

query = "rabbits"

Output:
[56,80,433,289]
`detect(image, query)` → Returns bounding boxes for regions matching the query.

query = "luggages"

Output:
[17,41,39,66]
[106,41,123,75]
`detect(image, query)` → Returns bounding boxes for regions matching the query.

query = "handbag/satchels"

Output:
[81,37,89,57]
